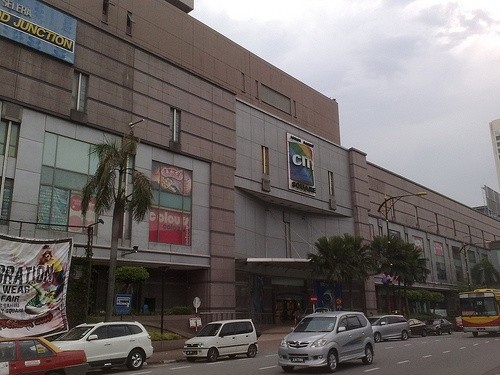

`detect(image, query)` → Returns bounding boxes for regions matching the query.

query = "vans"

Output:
[51,322,153,370]
[183,319,258,360]
[368,315,409,342]
[358,324,360,325]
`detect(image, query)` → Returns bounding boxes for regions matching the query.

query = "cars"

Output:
[0,337,88,372]
[455,315,463,330]
[407,318,428,336]
[425,319,453,333]
[278,309,374,372]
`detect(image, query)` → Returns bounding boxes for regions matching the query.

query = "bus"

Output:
[458,289,500,336]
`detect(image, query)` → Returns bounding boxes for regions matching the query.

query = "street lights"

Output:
[459,239,492,288]
[377,190,427,308]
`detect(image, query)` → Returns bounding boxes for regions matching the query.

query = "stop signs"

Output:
[308,295,319,304]
